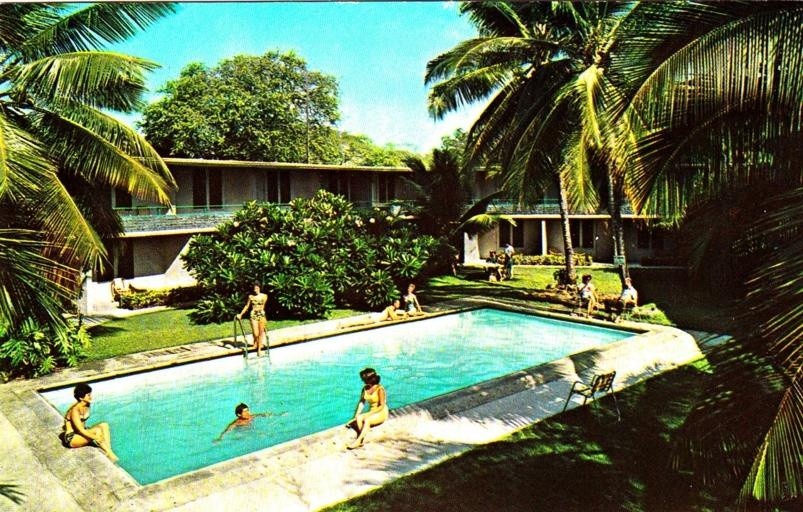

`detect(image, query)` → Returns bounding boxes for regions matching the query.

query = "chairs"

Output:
[562,368,622,426]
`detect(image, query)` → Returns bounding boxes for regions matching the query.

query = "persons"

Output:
[505,241,515,280]
[577,274,599,318]
[346,369,389,449]
[64,384,118,462]
[220,404,289,441]
[237,284,268,356]
[338,300,408,329]
[486,250,498,263]
[603,277,638,322]
[401,283,424,319]
